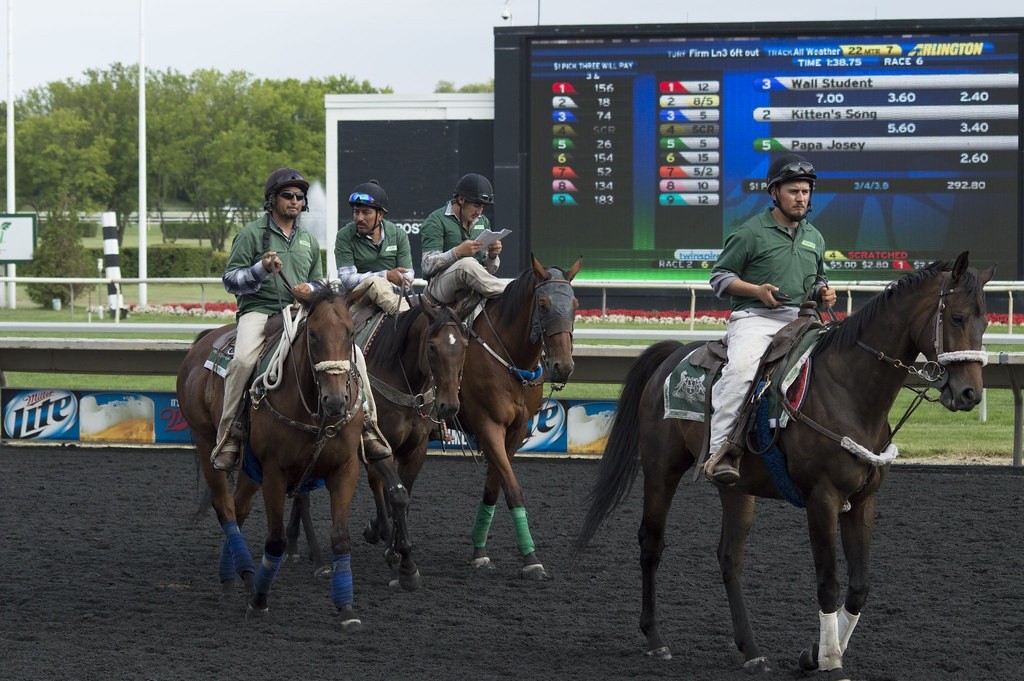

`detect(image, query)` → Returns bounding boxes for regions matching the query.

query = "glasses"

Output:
[481,195,496,202]
[780,161,814,177]
[348,192,381,205]
[279,190,304,200]
[277,173,304,183]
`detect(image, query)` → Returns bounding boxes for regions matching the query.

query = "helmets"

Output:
[264,168,310,212]
[453,174,496,204]
[348,179,390,213]
[766,153,817,195]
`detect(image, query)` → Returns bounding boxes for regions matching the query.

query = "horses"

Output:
[174,251,584,638]
[571,250,999,680]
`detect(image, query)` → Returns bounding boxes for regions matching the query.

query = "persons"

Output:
[212,167,326,468]
[334,179,415,313]
[705,154,838,486]
[421,171,519,306]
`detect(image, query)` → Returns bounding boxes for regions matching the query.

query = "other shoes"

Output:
[712,457,740,484]
[360,439,391,459]
[214,451,236,471]
